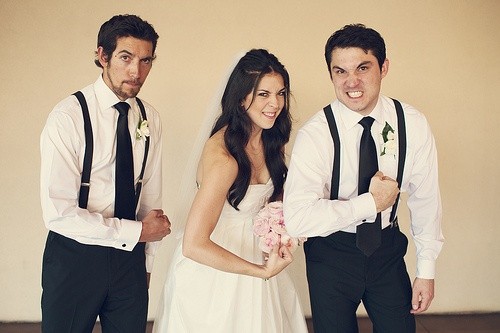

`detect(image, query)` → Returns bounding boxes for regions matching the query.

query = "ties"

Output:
[111,101,139,220]
[356,114,384,255]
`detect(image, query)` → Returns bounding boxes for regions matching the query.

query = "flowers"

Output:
[135,112,151,140]
[252,199,308,257]
[379,119,399,158]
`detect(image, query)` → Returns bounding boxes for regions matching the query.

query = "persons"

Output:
[279,21,448,332]
[154,45,310,333]
[39,14,173,333]
